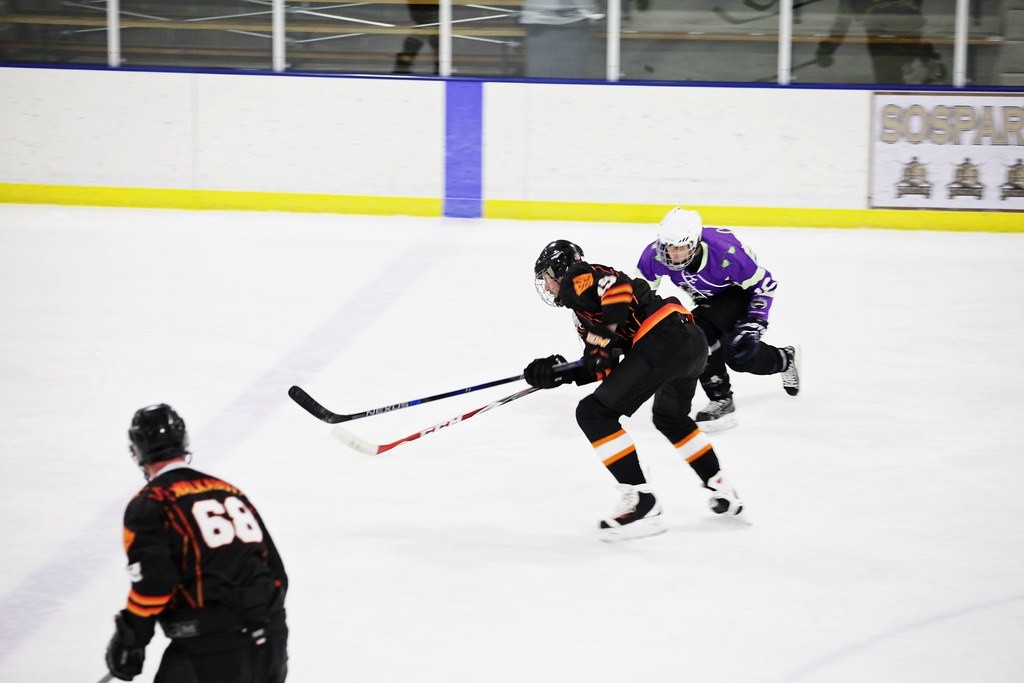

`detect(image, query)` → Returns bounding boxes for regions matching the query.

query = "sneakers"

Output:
[392,49,413,74]
[778,343,802,396]
[693,474,744,521]
[693,392,736,432]
[598,485,668,542]
[920,59,949,85]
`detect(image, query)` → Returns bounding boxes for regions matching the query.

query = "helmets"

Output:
[533,240,585,307]
[655,208,702,270]
[129,402,186,464]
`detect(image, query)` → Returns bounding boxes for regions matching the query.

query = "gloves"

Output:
[816,40,842,67]
[106,610,155,681]
[524,354,573,389]
[730,316,768,349]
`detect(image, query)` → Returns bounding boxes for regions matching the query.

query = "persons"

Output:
[817,0,1008,88]
[524,239,754,541]
[105,403,289,683]
[634,209,802,433]
[393,0,459,75]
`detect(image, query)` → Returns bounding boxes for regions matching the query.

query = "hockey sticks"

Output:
[286,356,602,426]
[329,376,563,456]
[98,651,127,683]
